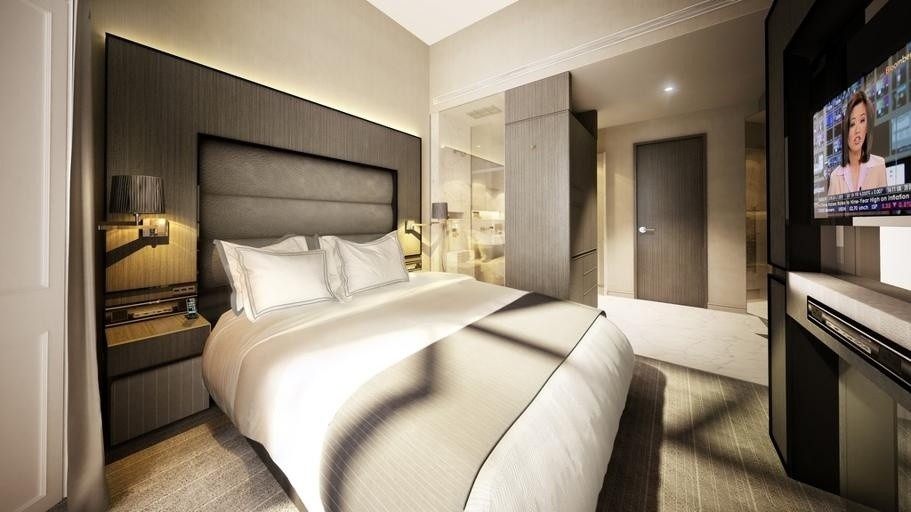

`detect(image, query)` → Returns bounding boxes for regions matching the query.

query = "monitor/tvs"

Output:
[805,38,911,225]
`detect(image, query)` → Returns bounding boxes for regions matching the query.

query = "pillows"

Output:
[212,228,411,324]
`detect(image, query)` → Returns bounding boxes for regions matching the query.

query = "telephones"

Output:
[186,298,199,319]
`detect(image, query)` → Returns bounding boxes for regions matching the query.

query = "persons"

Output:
[827,90,887,196]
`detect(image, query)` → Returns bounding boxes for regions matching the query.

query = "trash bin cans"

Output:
[446,250,476,277]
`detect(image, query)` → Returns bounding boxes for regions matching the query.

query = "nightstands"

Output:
[103,315,211,448]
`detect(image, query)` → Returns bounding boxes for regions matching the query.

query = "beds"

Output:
[186,138,634,512]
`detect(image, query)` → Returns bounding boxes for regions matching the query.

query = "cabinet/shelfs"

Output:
[504,75,599,312]
[758,259,910,512]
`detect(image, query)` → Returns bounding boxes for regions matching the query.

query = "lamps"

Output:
[405,201,449,231]
[97,173,169,239]
